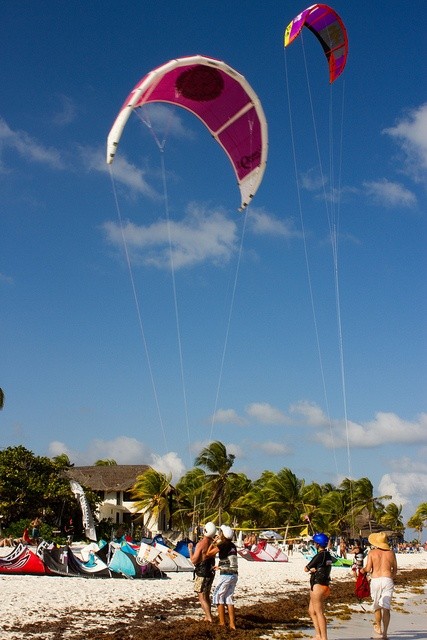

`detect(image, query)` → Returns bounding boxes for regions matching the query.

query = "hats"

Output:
[314,533,327,546]
[368,532,389,550]
[220,525,232,539]
[202,522,216,537]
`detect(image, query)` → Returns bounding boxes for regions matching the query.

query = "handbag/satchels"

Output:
[192,562,209,579]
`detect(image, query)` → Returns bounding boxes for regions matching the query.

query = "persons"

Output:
[30,517,42,545]
[409,541,414,555]
[400,543,403,552]
[360,532,397,640]
[64,519,74,546]
[304,533,332,640]
[252,533,256,546]
[351,541,364,580]
[287,536,294,556]
[404,541,407,554]
[339,539,347,558]
[398,541,400,554]
[0,535,14,548]
[352,563,357,577]
[249,537,252,547]
[207,525,238,629]
[244,533,248,546]
[188,522,216,622]
[415,540,421,552]
[22,527,34,546]
